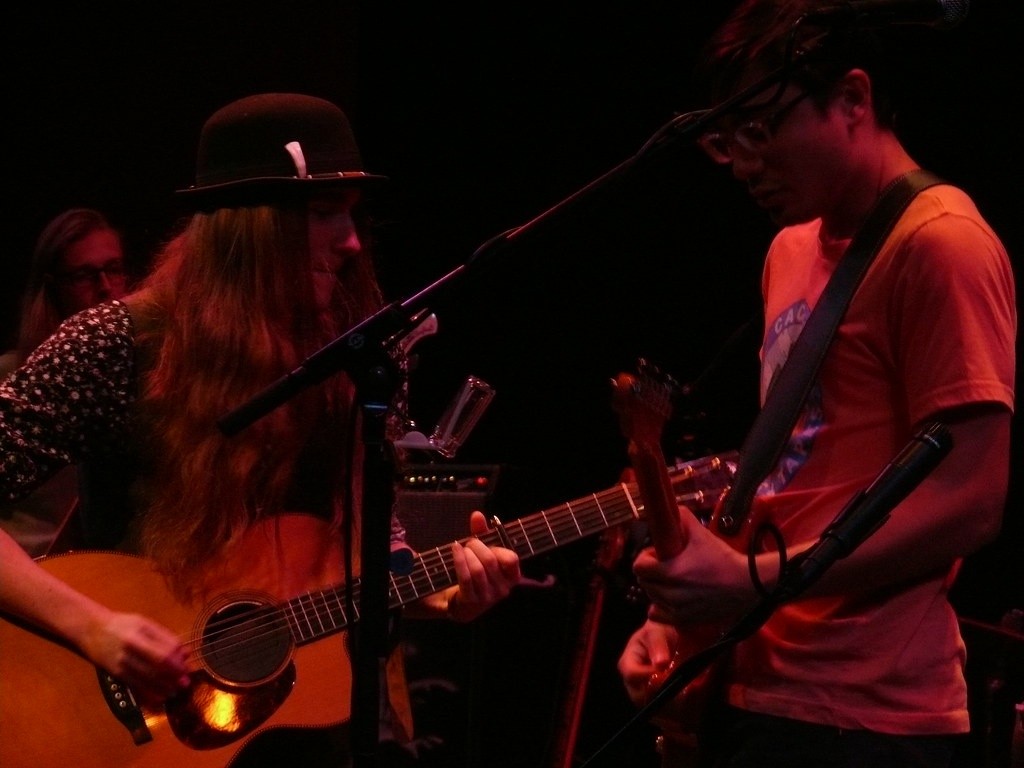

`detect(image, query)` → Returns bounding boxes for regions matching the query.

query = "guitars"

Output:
[608,363,767,742]
[0,451,749,766]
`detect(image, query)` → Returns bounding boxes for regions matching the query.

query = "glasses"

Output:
[697,90,813,166]
[53,262,129,289]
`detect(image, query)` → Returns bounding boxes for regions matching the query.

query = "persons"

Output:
[1,205,128,384]
[612,0,1021,768]
[1,89,555,711]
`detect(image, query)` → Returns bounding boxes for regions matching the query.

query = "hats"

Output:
[164,95,389,203]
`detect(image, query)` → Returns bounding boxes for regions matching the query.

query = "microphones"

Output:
[783,420,954,599]
[804,0,970,29]
